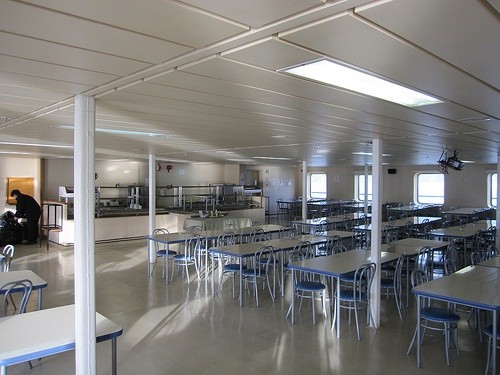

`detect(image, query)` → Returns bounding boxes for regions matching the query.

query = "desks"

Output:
[354,216,443,232]
[345,202,387,209]
[208,229,359,306]
[441,207,496,217]
[145,222,291,284]
[276,200,307,215]
[387,204,440,214]
[413,254,500,375]
[428,219,496,238]
[287,238,452,339]
[307,200,346,208]
[290,211,372,226]
[0,267,48,309]
[0,304,124,375]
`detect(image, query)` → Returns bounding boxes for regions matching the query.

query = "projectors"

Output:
[447,158,464,168]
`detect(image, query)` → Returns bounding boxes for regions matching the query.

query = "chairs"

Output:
[149,198,500,375]
[0,244,42,369]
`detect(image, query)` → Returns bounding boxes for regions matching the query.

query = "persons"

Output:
[11,190,42,245]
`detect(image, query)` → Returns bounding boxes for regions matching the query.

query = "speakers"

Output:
[388,169,396,174]
